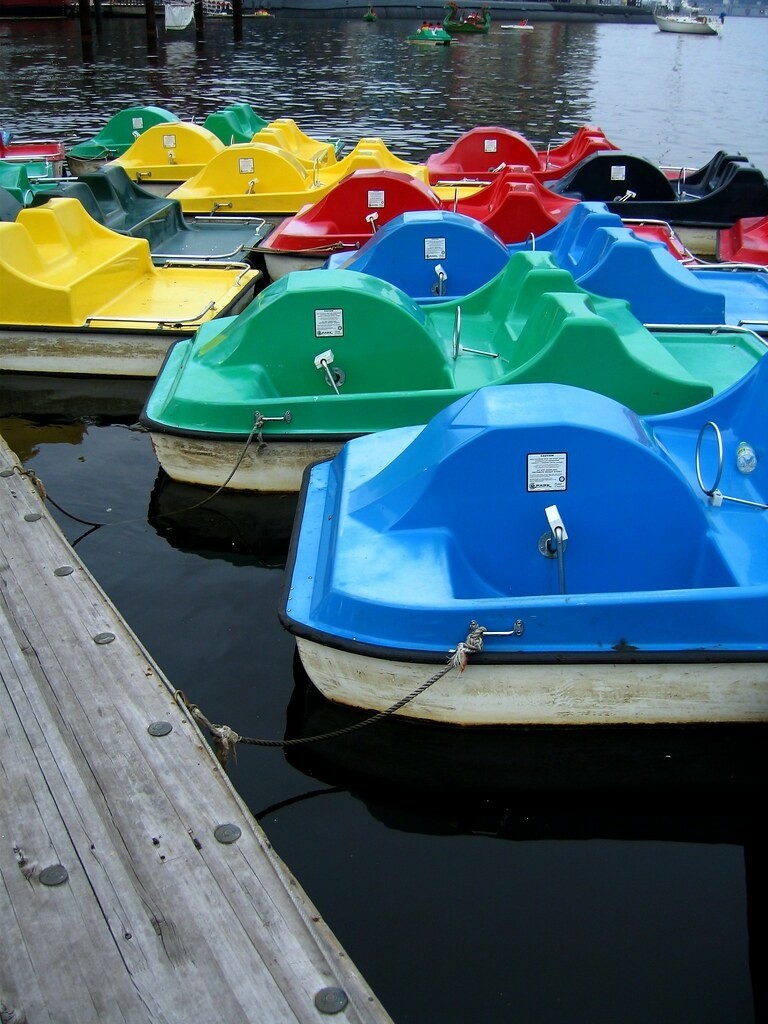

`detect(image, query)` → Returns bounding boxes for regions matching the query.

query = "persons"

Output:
[370,8,443,36]
[708,16,724,24]
[255,8,270,15]
[519,19,528,26]
[463,12,481,25]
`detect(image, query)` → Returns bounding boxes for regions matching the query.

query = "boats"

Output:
[652,0,725,36]
[402,23,453,48]
[361,4,378,23]
[1,197,263,341]
[309,199,768,353]
[499,24,536,31]
[278,344,768,730]
[141,252,767,494]
[443,0,492,33]
[1,97,768,278]
